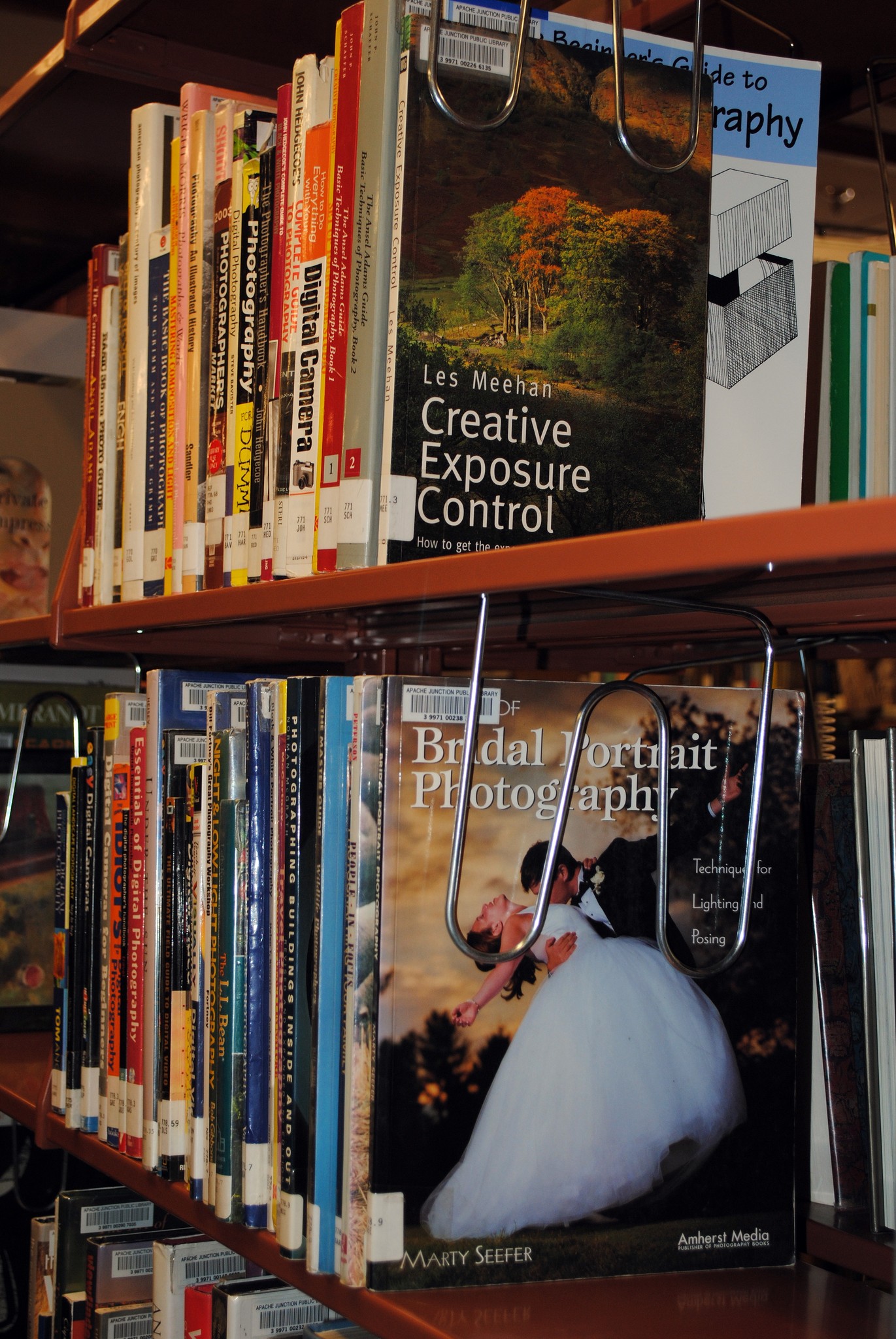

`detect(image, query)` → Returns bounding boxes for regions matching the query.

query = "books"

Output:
[4,0,896,1339]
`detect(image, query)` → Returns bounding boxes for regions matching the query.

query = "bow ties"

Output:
[573,880,590,904]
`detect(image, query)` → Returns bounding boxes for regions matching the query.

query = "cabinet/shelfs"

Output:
[0,0,896,1339]
